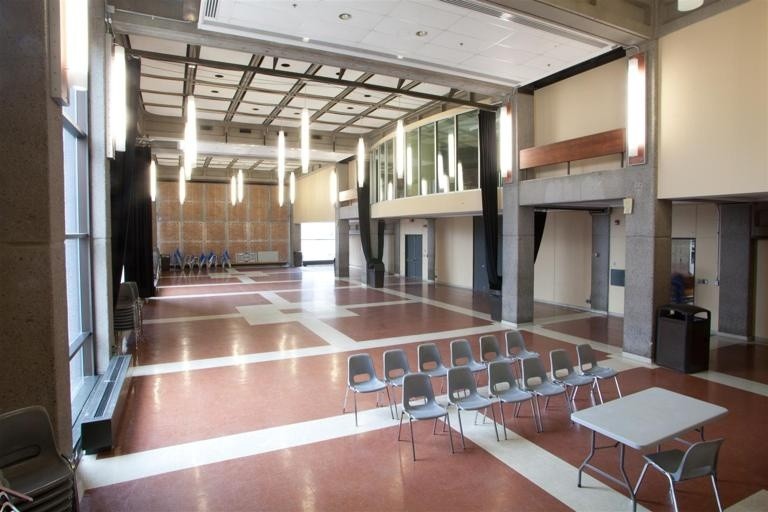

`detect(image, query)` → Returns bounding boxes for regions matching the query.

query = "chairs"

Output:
[344,330,625,461]
[112,282,146,352]
[632,436,725,511]
[0,405,78,512]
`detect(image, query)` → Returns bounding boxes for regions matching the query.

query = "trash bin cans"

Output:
[487,276,502,320]
[365,261,385,288]
[293,251,303,267]
[334,258,336,276]
[654,303,713,375]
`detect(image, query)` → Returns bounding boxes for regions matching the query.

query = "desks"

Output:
[570,386,722,511]
